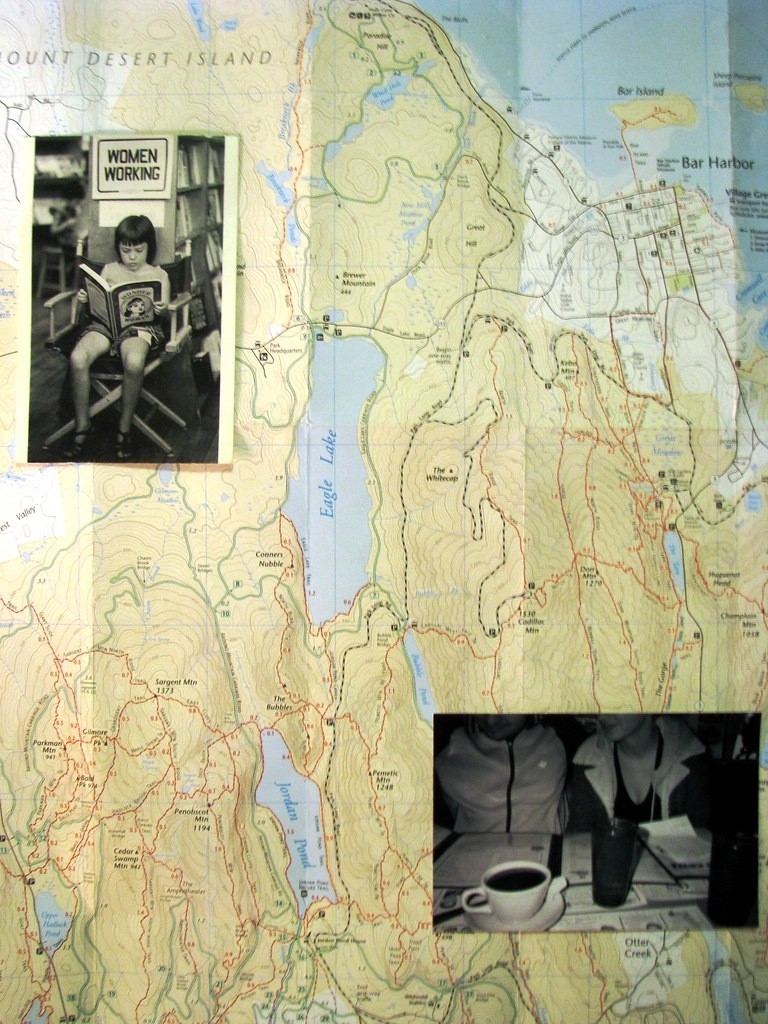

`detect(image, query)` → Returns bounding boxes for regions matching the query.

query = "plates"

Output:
[475,885,565,931]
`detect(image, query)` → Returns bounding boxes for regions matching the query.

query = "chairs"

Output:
[35,244,76,299]
[42,255,192,453]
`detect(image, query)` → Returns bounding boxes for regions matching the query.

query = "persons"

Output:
[51,205,79,263]
[434,715,717,835]
[62,214,170,462]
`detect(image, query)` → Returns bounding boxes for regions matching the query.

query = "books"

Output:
[34,155,84,226]
[80,143,224,397]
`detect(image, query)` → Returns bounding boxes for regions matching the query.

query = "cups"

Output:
[590,817,639,909]
[460,860,552,925]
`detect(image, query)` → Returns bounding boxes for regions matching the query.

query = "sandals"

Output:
[113,428,135,460]
[62,420,102,460]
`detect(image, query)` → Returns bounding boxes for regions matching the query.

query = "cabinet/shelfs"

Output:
[32,136,89,244]
[87,134,225,423]
